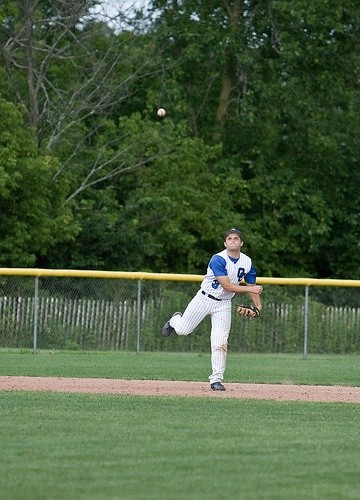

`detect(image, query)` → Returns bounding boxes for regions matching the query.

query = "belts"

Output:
[202,291,222,301]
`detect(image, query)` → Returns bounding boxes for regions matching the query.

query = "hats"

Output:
[224,228,243,240]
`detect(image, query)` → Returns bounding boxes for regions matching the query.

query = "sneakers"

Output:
[211,382,226,391]
[162,312,182,337]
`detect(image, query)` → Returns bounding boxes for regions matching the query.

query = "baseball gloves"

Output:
[235,303,261,318]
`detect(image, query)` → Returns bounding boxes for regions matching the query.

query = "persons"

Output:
[163,229,263,391]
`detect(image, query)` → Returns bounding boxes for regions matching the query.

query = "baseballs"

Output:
[157,108,166,117]
